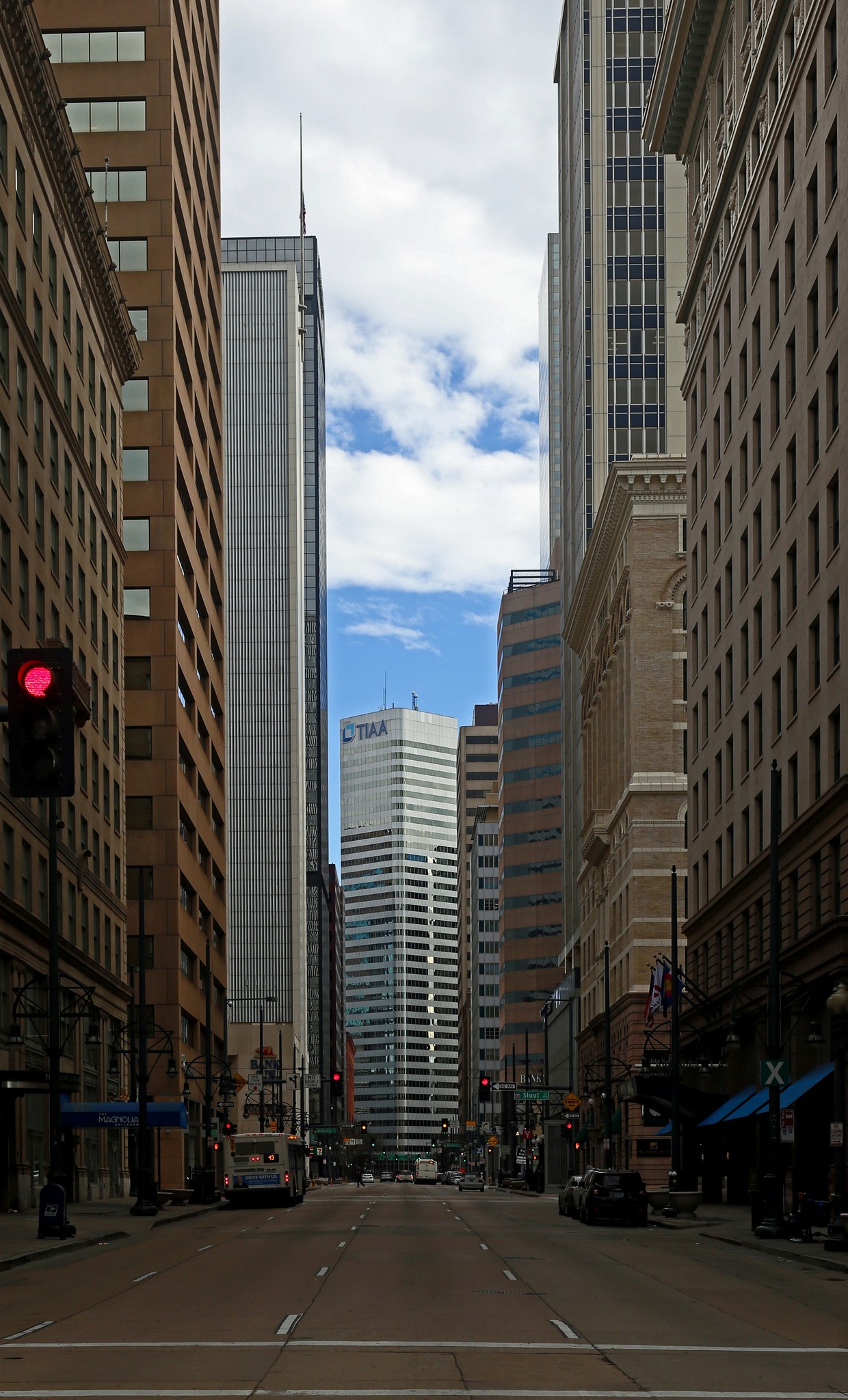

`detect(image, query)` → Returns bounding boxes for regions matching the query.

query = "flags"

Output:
[643,958,686,1028]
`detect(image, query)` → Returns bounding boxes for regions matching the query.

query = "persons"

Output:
[233,1176,249,1189]
[789,1189,827,1243]
[309,1170,315,1179]
[356,1167,366,1188]
[585,1160,594,1173]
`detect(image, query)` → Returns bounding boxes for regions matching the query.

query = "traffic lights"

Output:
[479,1077,490,1102]
[224,1122,238,1135]
[330,1072,344,1096]
[360,1116,493,1163]
[213,1142,223,1150]
[560,1122,573,1140]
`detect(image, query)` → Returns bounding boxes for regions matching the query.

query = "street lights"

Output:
[582,940,638,1169]
[4,796,102,1233]
[724,827,827,1229]
[210,996,278,1175]
[635,864,710,1190]
[181,939,235,1197]
[242,1004,282,1133]
[805,913,848,1251]
[107,876,179,1216]
[520,991,583,1178]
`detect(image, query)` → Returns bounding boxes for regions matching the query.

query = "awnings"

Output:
[654,1060,838,1136]
[573,1114,590,1142]
[600,1103,623,1135]
[59,1102,188,1129]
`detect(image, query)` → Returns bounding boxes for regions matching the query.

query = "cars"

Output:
[358,1156,485,1193]
[558,1168,648,1227]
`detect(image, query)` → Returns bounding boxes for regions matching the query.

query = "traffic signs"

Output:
[490,1081,518,1092]
[342,1123,355,1128]
[316,1129,336,1133]
[216,1100,234,1108]
[513,1090,549,1099]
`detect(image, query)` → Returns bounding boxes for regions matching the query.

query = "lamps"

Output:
[601,1093,606,1103]
[531,1134,545,1149]
[588,1099,594,1109]
[826,977,848,1017]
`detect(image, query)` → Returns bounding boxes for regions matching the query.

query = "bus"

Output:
[220,1134,312,1205]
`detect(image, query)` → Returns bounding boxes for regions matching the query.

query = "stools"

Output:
[801,1223,814,1243]
